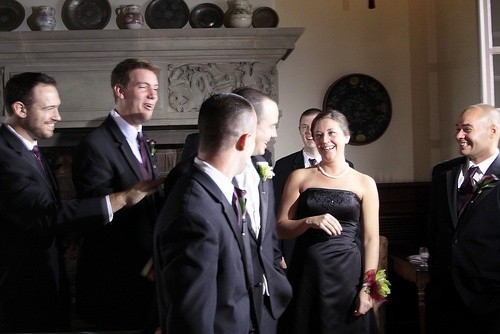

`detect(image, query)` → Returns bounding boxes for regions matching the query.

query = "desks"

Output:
[394,253,441,334]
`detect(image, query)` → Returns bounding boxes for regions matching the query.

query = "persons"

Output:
[0,72,157,334]
[154,93,262,334]
[425,104,500,334]
[276,110,379,334]
[74,58,355,334]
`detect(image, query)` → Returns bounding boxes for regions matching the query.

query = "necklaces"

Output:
[317,162,349,179]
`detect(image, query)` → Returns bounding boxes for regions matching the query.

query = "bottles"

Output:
[231,0,252,28]
[35,5,57,31]
[122,4,144,29]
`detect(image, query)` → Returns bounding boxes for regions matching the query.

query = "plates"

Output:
[61,0,112,31]
[189,3,225,29]
[144,0,190,29]
[0,0,25,32]
[252,7,279,29]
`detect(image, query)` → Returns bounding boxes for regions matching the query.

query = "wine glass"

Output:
[419,246,429,268]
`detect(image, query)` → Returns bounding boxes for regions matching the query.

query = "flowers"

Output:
[257,162,275,195]
[237,185,247,237]
[143,136,159,170]
[468,173,498,204]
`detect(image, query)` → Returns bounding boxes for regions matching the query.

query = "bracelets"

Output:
[362,269,391,302]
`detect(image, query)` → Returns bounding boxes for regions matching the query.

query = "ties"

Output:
[233,187,242,230]
[457,167,479,217]
[308,158,317,167]
[136,132,154,182]
[32,145,51,183]
